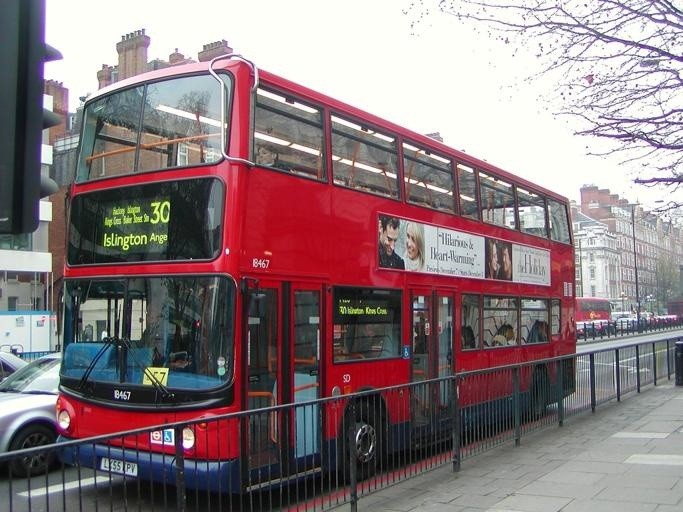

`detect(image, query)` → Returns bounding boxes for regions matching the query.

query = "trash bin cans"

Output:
[674,341,683,386]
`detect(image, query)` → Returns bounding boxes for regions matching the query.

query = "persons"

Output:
[378,214,405,269]
[136,299,181,363]
[497,245,512,280]
[257,143,288,171]
[402,221,425,271]
[346,318,549,354]
[488,239,497,279]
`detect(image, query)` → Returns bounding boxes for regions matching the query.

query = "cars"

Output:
[0,352,63,478]
[615,313,680,333]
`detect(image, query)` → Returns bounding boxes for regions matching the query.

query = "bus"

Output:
[576,297,614,337]
[54,53,577,496]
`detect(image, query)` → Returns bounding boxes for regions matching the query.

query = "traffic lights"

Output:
[0,0,62,234]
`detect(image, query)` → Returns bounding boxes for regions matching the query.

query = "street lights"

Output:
[604,204,642,334]
